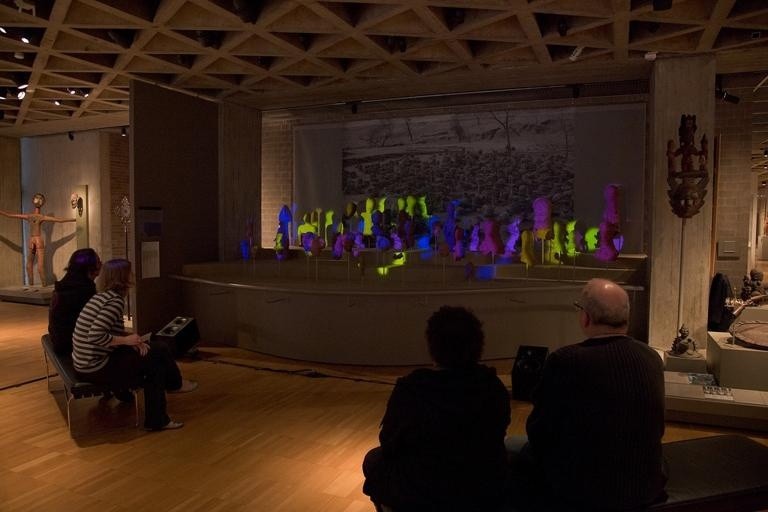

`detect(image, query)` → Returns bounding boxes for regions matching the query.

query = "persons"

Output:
[362,306,511,512]
[0,194,77,288]
[506,277,668,512]
[672,324,696,355]
[48,248,136,403]
[71,258,199,431]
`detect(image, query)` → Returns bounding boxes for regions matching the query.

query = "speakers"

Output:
[156,315,201,357]
[511,345,548,401]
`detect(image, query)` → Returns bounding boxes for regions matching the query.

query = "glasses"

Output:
[574,299,586,314]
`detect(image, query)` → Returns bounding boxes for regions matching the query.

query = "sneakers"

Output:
[167,379,200,393]
[147,421,187,430]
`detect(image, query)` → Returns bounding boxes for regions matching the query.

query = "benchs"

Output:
[371,432,766,512]
[40,333,140,440]
